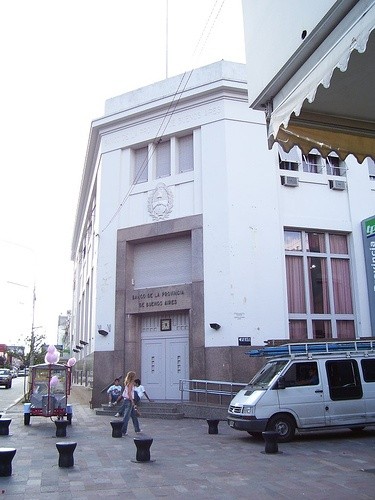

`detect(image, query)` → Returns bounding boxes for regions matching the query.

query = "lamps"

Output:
[76,346,83,349]
[209,323,220,330]
[80,340,88,345]
[72,349,79,353]
[98,329,108,336]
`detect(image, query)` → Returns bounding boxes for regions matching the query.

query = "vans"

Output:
[225,339,375,444]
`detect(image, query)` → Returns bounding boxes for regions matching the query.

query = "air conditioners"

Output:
[282,176,299,187]
[329,180,346,190]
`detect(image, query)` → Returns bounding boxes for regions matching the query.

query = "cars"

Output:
[10,367,30,378]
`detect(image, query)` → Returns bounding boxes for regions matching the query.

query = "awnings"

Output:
[267,0,375,164]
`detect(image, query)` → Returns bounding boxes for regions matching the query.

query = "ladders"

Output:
[245,342,375,356]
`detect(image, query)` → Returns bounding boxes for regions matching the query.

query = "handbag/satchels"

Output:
[136,409,142,416]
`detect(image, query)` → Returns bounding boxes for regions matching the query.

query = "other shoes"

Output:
[108,402,112,407]
[134,405,137,410]
[115,412,120,417]
[112,401,117,406]
[122,432,128,436]
[136,432,143,434]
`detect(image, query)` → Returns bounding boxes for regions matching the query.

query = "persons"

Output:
[279,364,319,386]
[122,370,144,436]
[114,379,155,417]
[107,378,123,407]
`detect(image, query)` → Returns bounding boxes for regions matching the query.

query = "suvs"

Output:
[0,369,13,389]
